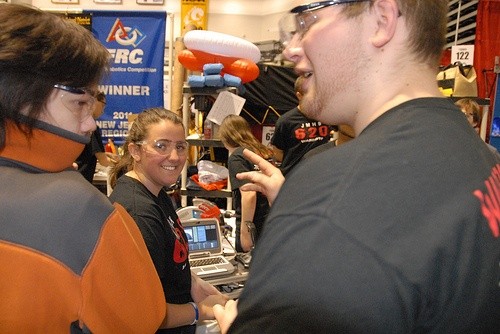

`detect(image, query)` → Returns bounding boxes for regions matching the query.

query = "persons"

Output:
[271,74,337,176]
[454,98,499,161]
[300,124,354,161]
[107,108,230,334]
[0,4,166,334]
[220,116,276,253]
[214,0,500,334]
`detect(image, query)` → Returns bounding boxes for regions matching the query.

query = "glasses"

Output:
[135,138,188,153]
[290,0,365,29]
[53,84,105,116]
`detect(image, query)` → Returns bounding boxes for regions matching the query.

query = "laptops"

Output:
[180,218,235,279]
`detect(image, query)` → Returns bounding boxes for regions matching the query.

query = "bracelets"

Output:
[188,302,199,325]
